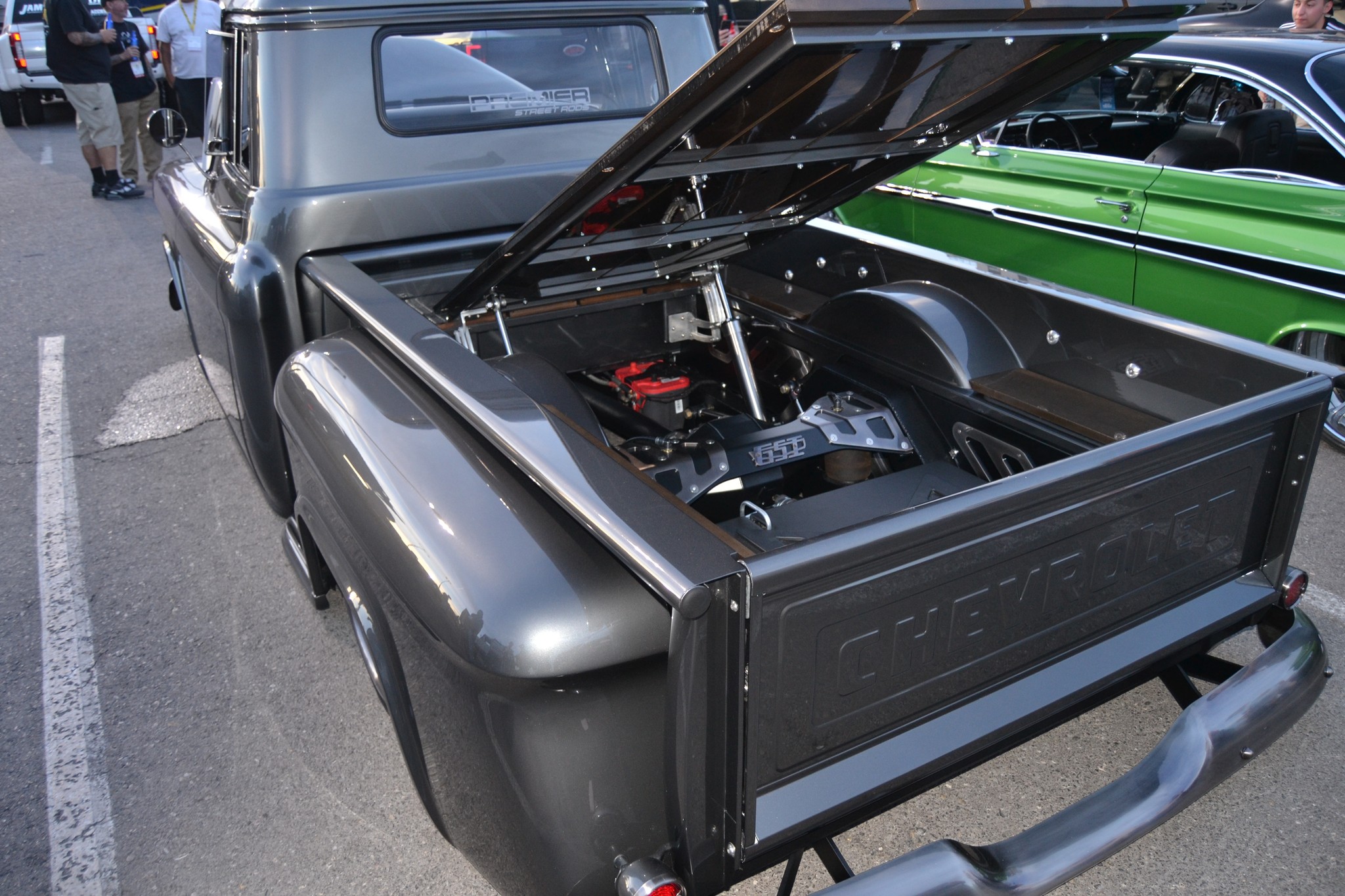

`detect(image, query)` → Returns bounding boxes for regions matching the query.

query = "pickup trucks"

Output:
[145,0,1345,896]
[0,0,166,126]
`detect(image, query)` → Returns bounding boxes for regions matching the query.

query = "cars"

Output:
[825,24,1344,454]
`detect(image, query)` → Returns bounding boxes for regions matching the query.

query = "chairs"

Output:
[1214,107,1297,164]
[1147,134,1240,167]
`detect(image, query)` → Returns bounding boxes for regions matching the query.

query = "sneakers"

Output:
[104,182,144,201]
[124,178,137,189]
[91,182,110,197]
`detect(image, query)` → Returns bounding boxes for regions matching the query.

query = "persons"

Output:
[1257,0,1345,131]
[98,0,164,184]
[154,0,223,139]
[44,0,146,199]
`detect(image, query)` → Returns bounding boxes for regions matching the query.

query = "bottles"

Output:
[130,30,140,61]
[104,12,114,30]
